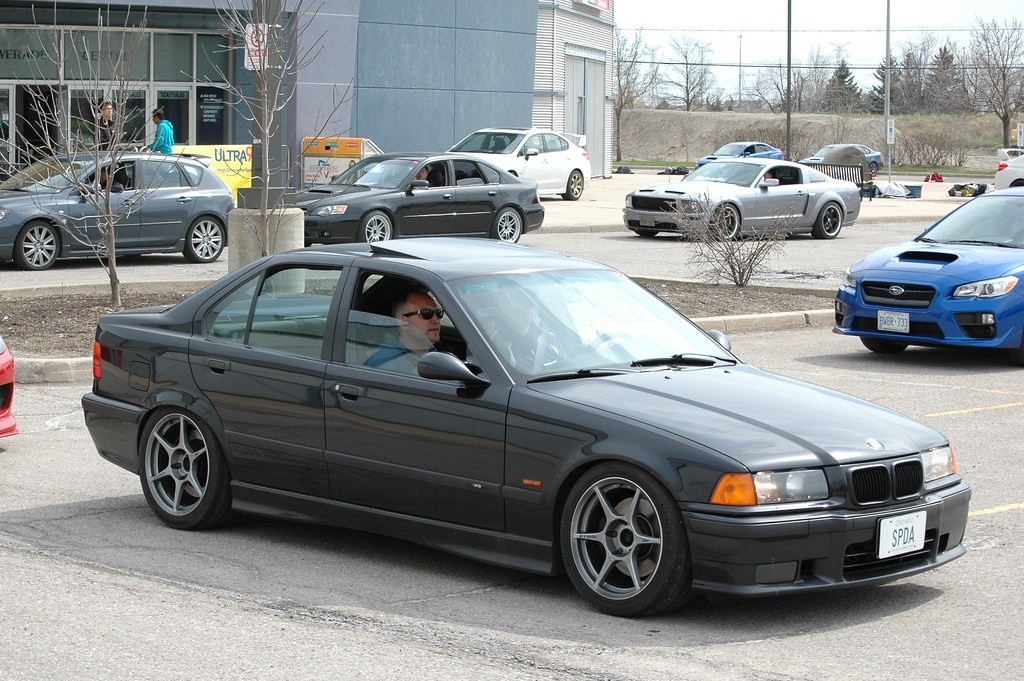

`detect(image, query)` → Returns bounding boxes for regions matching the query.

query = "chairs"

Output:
[427,167,468,186]
[114,168,128,186]
[464,288,499,331]
[491,138,506,151]
[773,168,783,185]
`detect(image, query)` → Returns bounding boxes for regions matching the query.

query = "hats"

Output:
[153,109,164,115]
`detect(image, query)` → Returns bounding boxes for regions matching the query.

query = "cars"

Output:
[797,144,882,178]
[445,128,591,201]
[995,148,1024,189]
[0,153,234,270]
[831,187,1024,367]
[621,157,861,242]
[81,236,972,618]
[696,141,785,170]
[290,152,546,249]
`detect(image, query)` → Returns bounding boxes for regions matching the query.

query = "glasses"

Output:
[402,309,445,320]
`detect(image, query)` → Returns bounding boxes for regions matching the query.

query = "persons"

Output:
[95,166,123,193]
[142,108,174,155]
[762,170,773,179]
[416,166,429,180]
[363,291,441,375]
[92,102,116,151]
[474,290,569,377]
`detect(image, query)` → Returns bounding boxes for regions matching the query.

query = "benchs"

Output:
[217,317,328,360]
